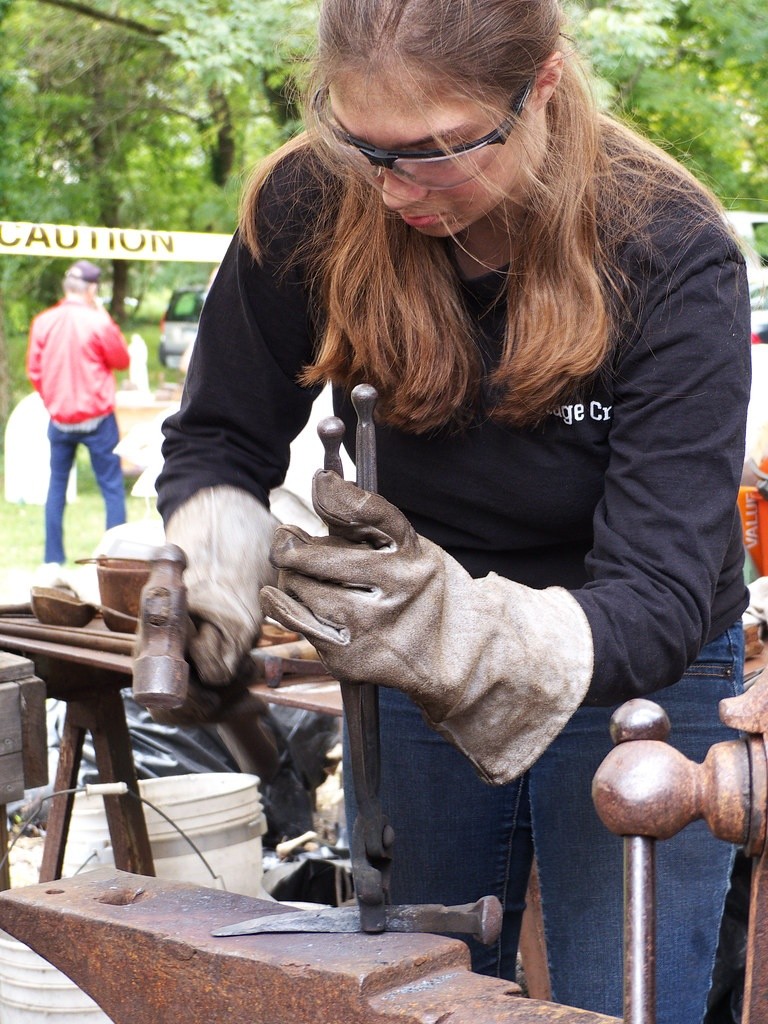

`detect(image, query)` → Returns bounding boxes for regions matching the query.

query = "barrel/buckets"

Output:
[61,772,267,899]
[0,782,226,1024]
[75,554,153,634]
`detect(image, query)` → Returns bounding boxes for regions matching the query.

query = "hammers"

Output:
[134,543,281,783]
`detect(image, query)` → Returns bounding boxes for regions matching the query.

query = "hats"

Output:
[68,260,101,283]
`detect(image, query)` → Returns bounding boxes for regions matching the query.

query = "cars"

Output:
[722,208,767,343]
[157,285,208,367]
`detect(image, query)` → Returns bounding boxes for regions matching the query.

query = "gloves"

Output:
[164,485,284,686]
[259,469,594,787]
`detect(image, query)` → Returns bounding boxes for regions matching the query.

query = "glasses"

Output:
[311,72,535,190]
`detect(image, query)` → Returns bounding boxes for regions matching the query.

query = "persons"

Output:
[157,0,751,1024]
[26,259,131,567]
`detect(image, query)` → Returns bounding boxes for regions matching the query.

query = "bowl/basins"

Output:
[30,584,99,628]
